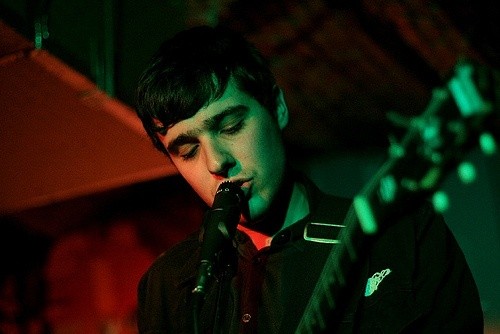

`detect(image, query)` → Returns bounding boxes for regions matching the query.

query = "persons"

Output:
[136,23,485,334]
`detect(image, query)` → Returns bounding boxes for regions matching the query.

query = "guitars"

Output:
[295,55,500,334]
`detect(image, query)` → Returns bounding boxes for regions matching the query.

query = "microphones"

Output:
[190,181,242,296]
